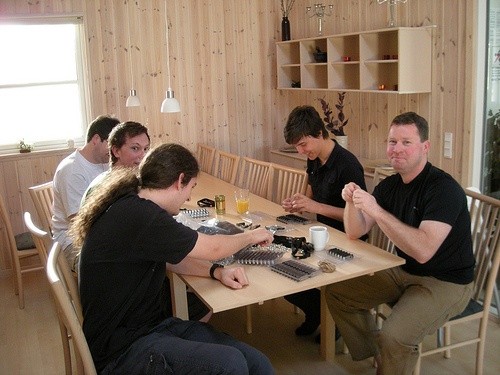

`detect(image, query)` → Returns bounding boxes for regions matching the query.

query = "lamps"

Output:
[305,3,333,36]
[125,0,142,108]
[161,0,181,113]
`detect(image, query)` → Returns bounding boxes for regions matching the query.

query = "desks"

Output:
[271,149,385,194]
[167,171,406,363]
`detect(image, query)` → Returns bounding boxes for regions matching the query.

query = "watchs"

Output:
[209,263,224,280]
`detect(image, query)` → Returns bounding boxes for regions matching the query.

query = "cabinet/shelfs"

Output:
[275,26,433,94]
[0,148,78,235]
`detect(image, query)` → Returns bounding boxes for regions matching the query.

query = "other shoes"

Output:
[373,329,383,366]
[312,325,342,345]
[295,319,320,337]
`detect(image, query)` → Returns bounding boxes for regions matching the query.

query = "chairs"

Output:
[198,150,500,375]
[0,181,97,375]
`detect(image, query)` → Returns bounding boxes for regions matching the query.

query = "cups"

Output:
[234,189,249,214]
[383,48,398,60]
[309,225,329,251]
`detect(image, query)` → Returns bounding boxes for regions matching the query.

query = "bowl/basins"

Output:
[312,51,327,62]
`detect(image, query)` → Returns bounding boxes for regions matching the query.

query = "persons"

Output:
[282,105,368,344]
[71,142,275,375]
[80,120,150,245]
[53,114,121,274]
[326,112,475,375]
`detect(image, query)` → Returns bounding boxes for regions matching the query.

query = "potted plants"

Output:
[18,139,34,153]
[280,0,294,41]
[314,91,349,149]
